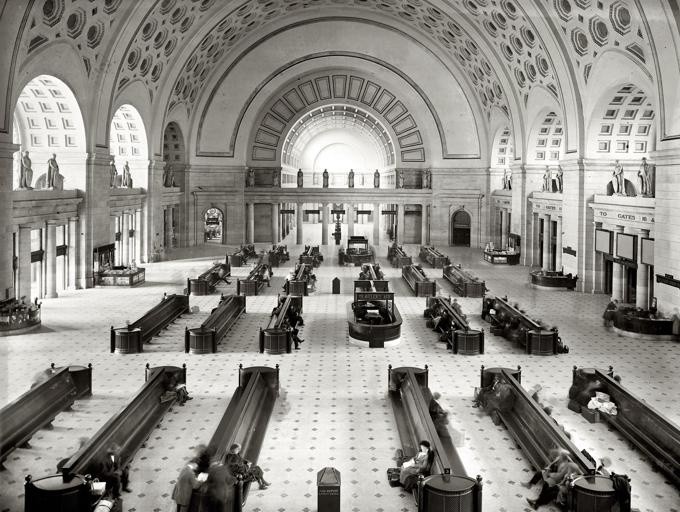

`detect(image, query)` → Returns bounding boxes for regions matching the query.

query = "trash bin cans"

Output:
[317,467,341,512]
[332,277,340,294]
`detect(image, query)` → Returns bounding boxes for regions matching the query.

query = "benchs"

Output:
[568,365,679,496]
[23,360,188,511]
[385,363,485,511]
[174,361,281,511]
[0,359,93,476]
[470,365,632,511]
[109,246,570,356]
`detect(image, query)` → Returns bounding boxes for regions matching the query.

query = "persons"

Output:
[504,169,512,190]
[556,165,563,193]
[237,245,255,265]
[425,297,470,349]
[485,379,514,411]
[386,440,431,491]
[121,160,131,187]
[612,160,623,194]
[525,462,580,510]
[270,298,305,350]
[638,157,649,194]
[110,159,117,188]
[481,296,567,354]
[103,441,132,498]
[358,265,370,280]
[520,449,569,489]
[224,443,273,490]
[470,374,499,410]
[164,374,193,406]
[416,264,426,277]
[283,264,317,294]
[48,152,59,188]
[217,267,232,285]
[170,458,210,512]
[259,264,271,288]
[374,264,385,280]
[602,299,618,328]
[21,150,34,190]
[613,374,622,384]
[207,458,242,512]
[542,165,552,192]
